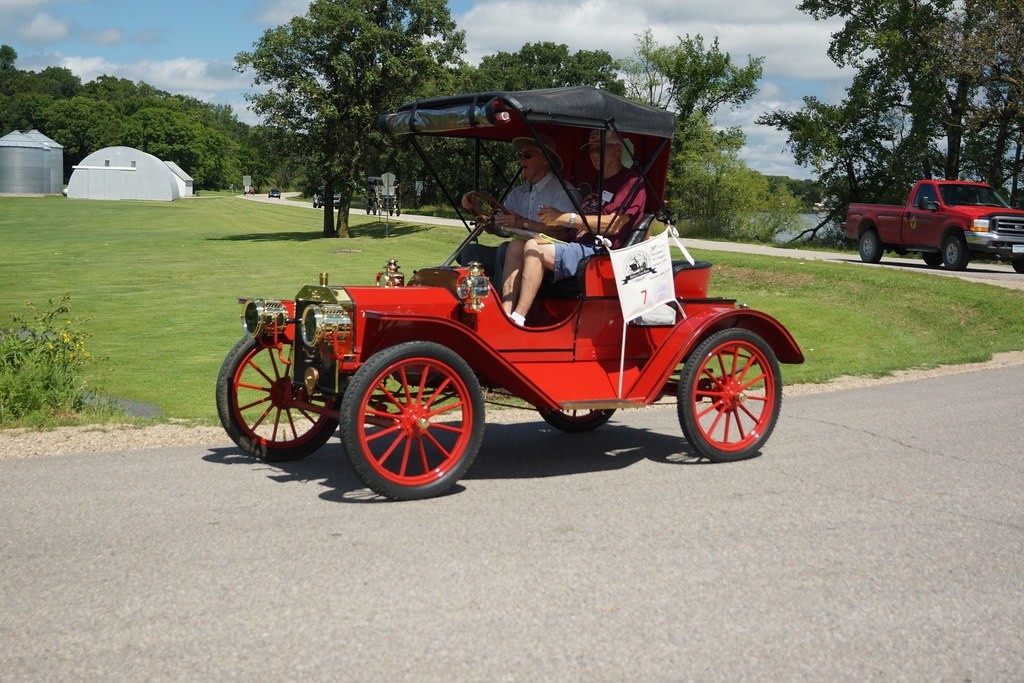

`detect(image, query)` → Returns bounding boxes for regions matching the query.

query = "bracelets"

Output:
[565,212,577,228]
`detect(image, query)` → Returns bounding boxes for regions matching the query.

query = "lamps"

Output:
[384,259,404,286]
[463,261,490,314]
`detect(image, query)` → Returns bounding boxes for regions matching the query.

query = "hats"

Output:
[512,130,564,172]
[580,128,623,153]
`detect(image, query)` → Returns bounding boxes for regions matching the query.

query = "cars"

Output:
[215,85,805,502]
[366,176,402,216]
[312,185,342,210]
[268,189,281,199]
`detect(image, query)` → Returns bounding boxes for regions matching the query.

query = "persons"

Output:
[249,184,255,196]
[456,135,584,295]
[503,128,646,327]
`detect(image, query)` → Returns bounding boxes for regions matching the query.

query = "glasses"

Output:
[515,150,531,159]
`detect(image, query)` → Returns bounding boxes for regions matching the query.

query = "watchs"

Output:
[523,217,529,230]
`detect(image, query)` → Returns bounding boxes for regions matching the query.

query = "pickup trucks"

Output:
[842,179,1024,271]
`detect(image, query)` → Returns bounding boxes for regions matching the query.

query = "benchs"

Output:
[538,214,654,299]
